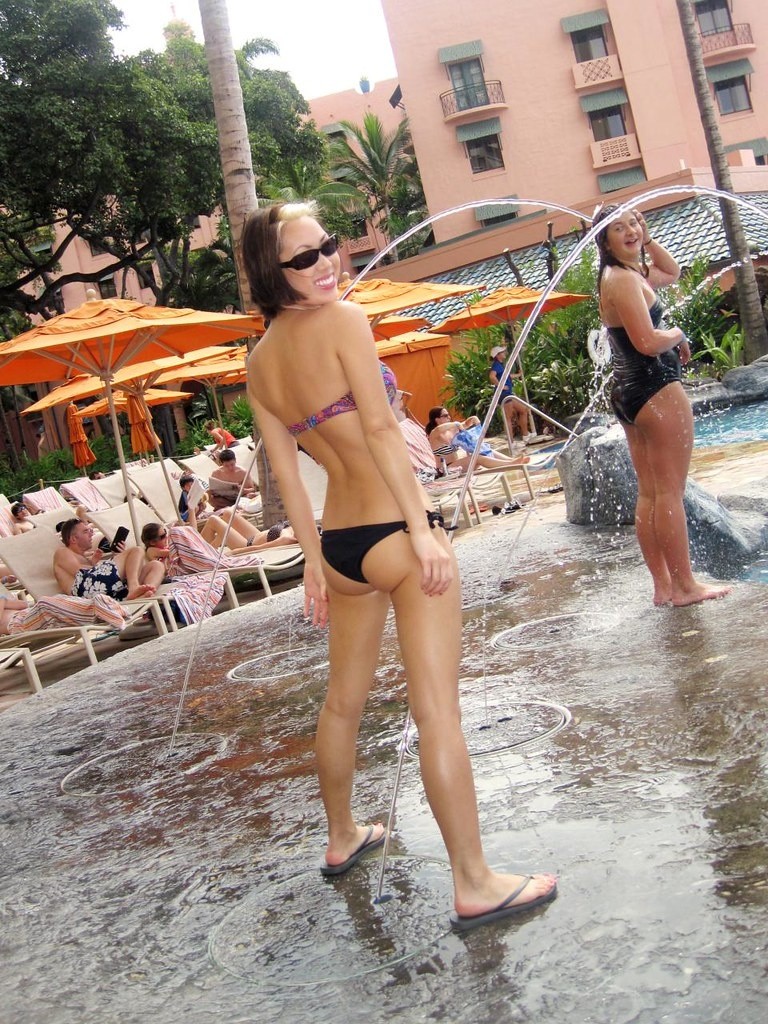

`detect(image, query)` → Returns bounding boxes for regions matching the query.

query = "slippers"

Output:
[320,823,387,874]
[450,874,558,928]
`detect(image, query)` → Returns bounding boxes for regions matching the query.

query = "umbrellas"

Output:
[19,343,247,528]
[427,284,592,447]
[336,271,485,316]
[371,316,451,358]
[0,290,258,565]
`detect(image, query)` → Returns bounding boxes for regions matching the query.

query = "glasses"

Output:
[440,413,450,418]
[278,234,338,271]
[17,506,25,512]
[154,529,166,540]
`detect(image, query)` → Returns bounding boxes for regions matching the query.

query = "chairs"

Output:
[1,433,535,695]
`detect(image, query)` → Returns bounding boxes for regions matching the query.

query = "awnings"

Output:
[390,86,404,109]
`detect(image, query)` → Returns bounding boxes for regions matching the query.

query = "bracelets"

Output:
[646,235,654,246]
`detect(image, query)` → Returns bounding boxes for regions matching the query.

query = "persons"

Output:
[489,346,537,443]
[140,523,228,573]
[179,477,205,520]
[204,422,240,453]
[89,470,148,505]
[423,404,531,474]
[10,504,42,534]
[53,519,165,598]
[0,567,126,634]
[210,449,254,509]
[391,391,411,422]
[199,509,323,556]
[242,199,558,930]
[592,202,730,606]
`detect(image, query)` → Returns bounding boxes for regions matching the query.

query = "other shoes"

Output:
[523,432,537,441]
[511,441,526,448]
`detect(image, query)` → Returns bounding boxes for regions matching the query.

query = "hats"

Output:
[491,346,507,358]
